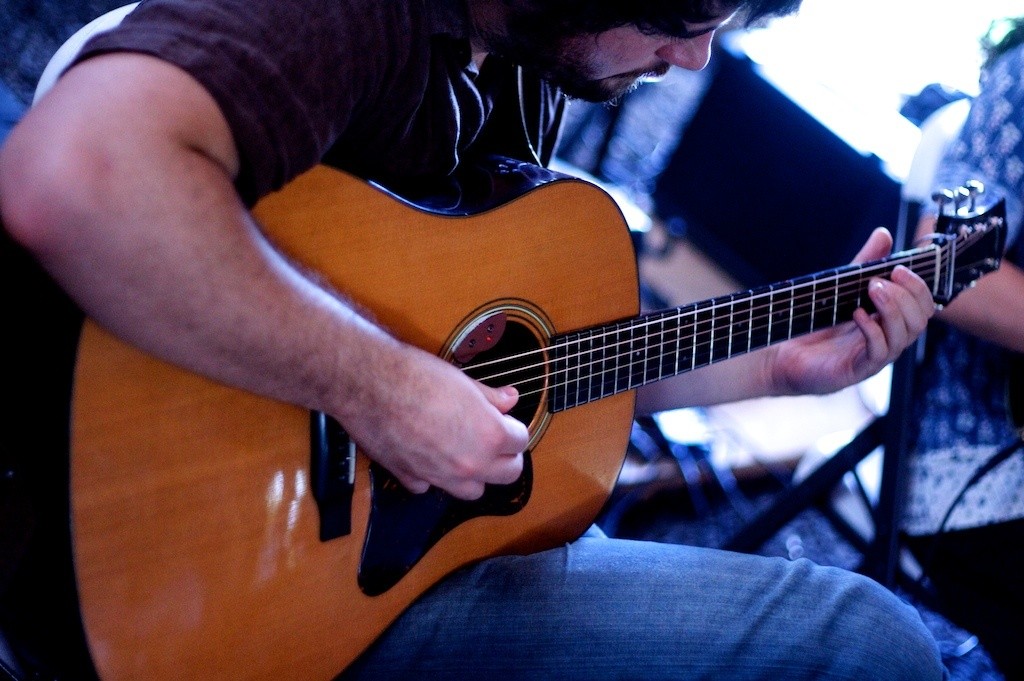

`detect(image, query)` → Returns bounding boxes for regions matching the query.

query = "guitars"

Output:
[1,152,1011,680]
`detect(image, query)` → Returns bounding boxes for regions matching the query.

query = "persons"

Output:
[1,1,952,679]
[899,40,1024,681]
